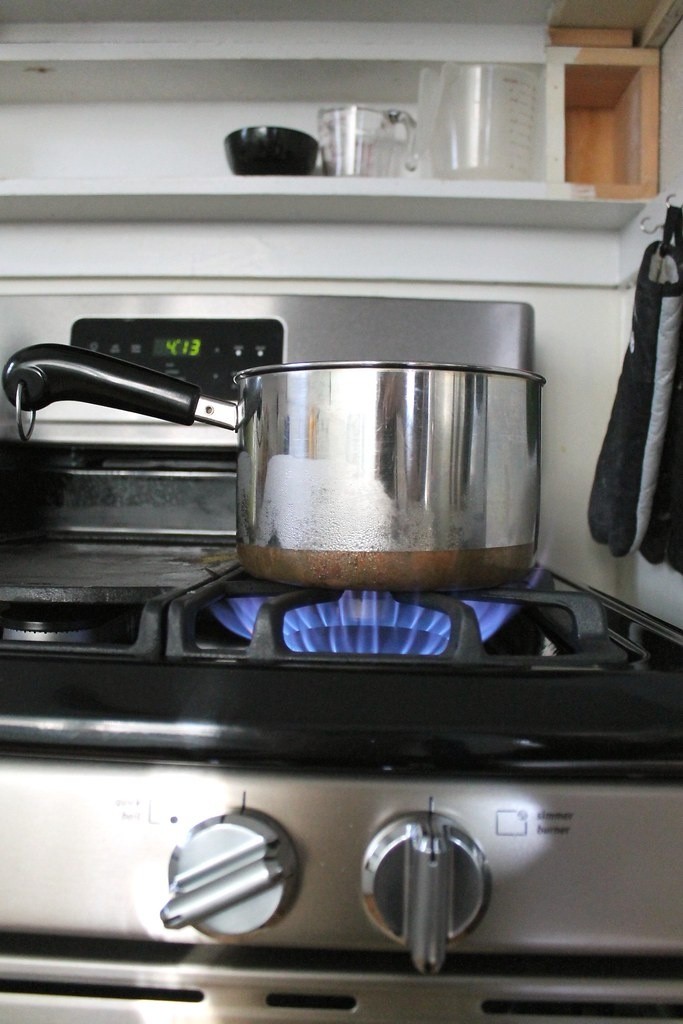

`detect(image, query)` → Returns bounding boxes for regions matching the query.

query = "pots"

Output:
[3,342,548,597]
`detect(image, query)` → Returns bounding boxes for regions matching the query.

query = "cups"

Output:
[319,105,420,180]
[428,62,546,181]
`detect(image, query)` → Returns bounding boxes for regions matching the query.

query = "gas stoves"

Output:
[0,524,683,787]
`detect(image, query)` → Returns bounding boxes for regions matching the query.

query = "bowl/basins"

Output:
[222,126,319,176]
